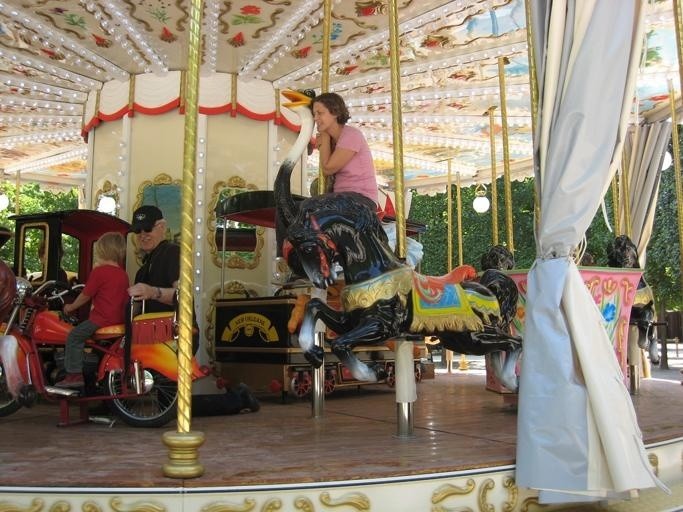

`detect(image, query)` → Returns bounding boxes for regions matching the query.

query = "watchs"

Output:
[151,286,162,299]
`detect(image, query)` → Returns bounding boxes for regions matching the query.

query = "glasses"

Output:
[134,228,151,233]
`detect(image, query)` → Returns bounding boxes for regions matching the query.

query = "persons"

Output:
[33,239,68,282]
[310,92,378,211]
[54,231,129,388]
[126,205,180,314]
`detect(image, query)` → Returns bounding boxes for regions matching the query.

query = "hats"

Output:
[128,205,162,231]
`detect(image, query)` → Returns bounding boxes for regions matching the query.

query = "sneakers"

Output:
[55,373,85,388]
